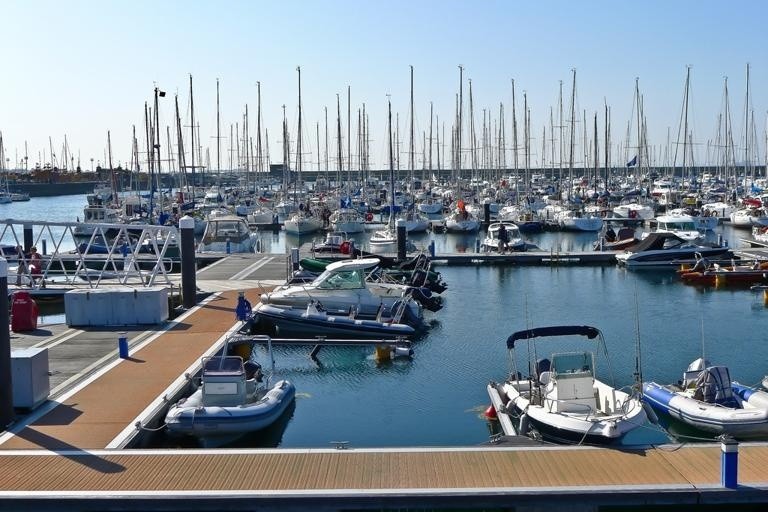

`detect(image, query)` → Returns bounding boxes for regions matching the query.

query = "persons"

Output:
[27,246,41,287]
[497,224,514,256]
[15,245,36,285]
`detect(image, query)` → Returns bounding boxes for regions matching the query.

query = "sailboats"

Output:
[0,56,768,258]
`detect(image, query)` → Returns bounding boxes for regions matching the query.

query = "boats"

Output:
[485,269,659,442]
[480,221,527,252]
[639,308,768,439]
[165,334,294,450]
[196,214,265,254]
[672,253,766,290]
[256,231,450,342]
[613,231,742,267]
